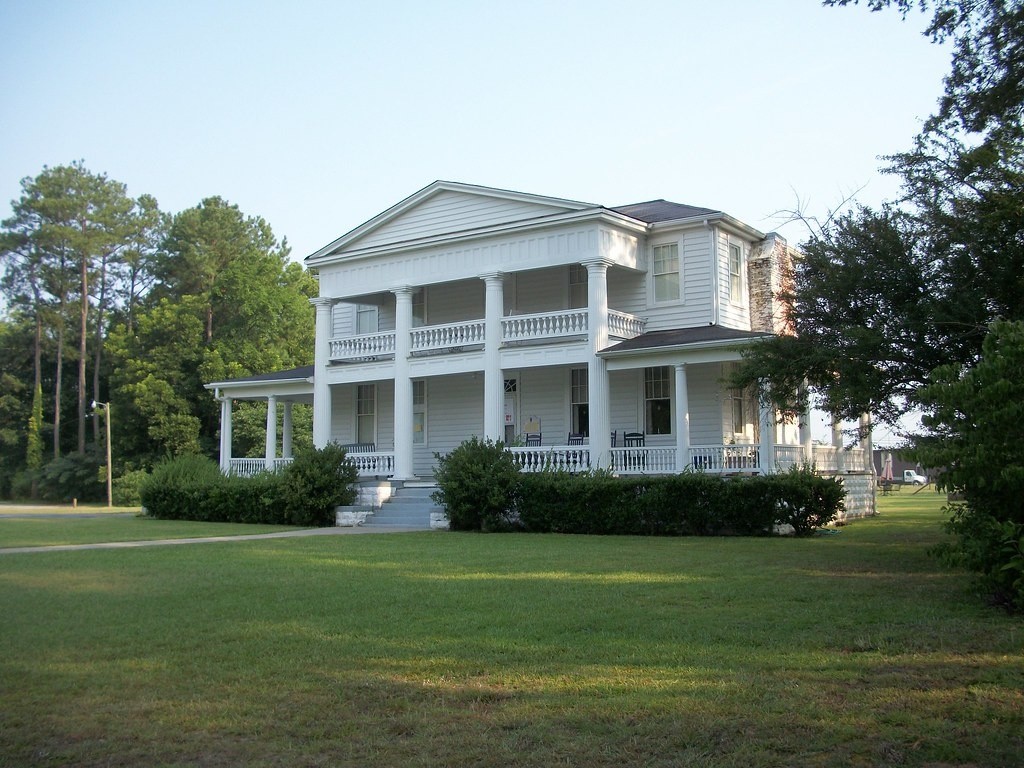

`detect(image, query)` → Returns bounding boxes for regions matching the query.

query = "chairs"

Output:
[522,432,542,467]
[623,431,648,470]
[554,433,584,469]
[587,431,616,469]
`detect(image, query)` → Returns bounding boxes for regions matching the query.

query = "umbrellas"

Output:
[881,453,894,481]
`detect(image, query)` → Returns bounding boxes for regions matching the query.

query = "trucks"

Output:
[892,469,926,486]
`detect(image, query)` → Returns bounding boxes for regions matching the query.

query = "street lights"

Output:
[91,399,112,507]
